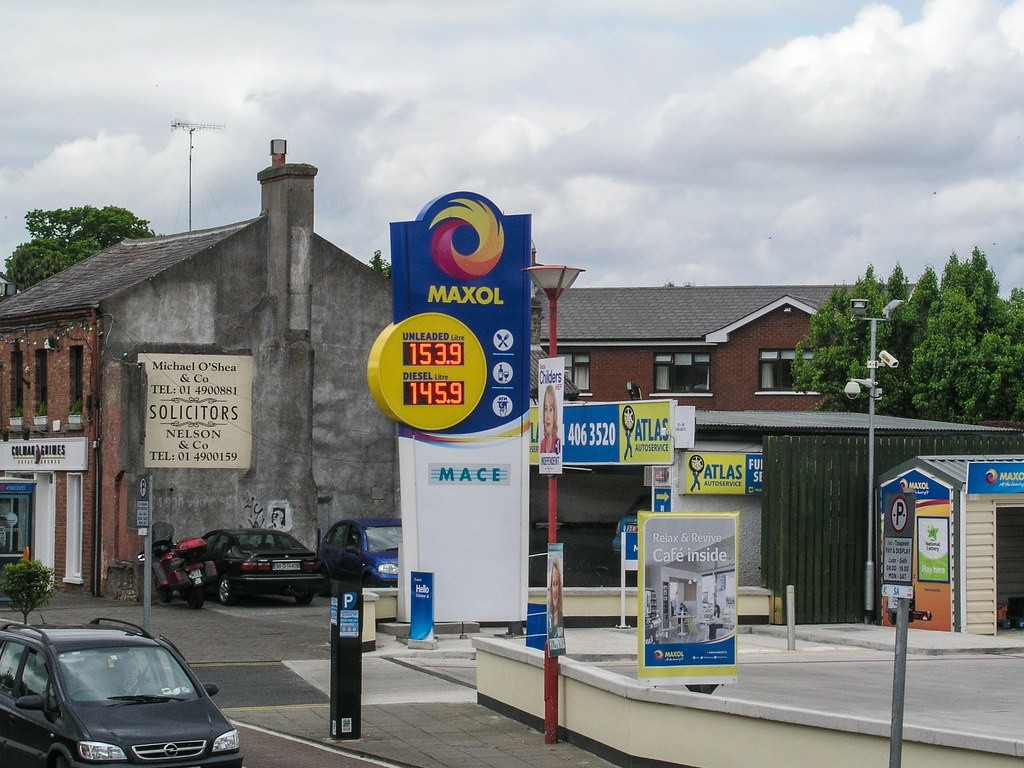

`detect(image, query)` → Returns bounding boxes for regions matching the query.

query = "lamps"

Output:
[626,381,642,400]
[269,139,287,155]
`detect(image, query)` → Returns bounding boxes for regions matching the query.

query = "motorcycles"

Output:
[151,522,206,610]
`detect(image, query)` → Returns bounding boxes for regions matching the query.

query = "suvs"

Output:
[1,616,243,768]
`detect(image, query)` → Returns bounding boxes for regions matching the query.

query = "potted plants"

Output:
[32,401,48,425]
[8,406,24,425]
[67,397,83,423]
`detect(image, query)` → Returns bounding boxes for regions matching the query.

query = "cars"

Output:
[201,529,324,606]
[319,517,400,586]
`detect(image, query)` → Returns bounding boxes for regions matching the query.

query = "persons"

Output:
[709,605,724,639]
[678,603,687,623]
[540,385,560,452]
[548,561,564,638]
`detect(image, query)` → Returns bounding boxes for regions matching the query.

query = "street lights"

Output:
[845,299,905,624]
[523,265,585,742]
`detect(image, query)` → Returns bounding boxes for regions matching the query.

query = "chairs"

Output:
[221,543,289,551]
[12,651,126,697]
